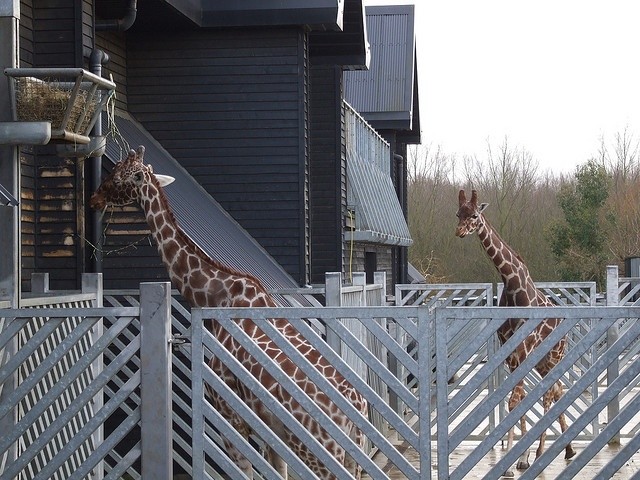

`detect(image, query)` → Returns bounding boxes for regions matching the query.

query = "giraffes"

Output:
[454,188,576,477]
[88,143,369,480]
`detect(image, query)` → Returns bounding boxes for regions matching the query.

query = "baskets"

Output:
[5,67,117,145]
